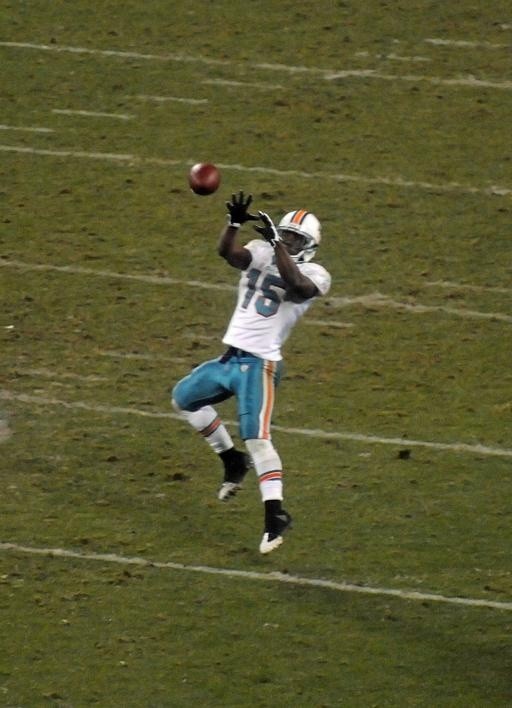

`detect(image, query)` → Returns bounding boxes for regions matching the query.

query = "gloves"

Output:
[226,190,284,247]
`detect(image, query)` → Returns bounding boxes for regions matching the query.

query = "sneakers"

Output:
[218,452,255,501]
[259,511,292,555]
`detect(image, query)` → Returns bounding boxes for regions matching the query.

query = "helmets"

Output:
[278,209,323,263]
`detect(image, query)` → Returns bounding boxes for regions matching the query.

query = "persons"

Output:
[171,190,333,556]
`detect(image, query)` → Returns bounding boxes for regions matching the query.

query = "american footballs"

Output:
[190,162,220,195]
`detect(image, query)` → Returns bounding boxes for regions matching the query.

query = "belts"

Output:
[219,347,246,364]
